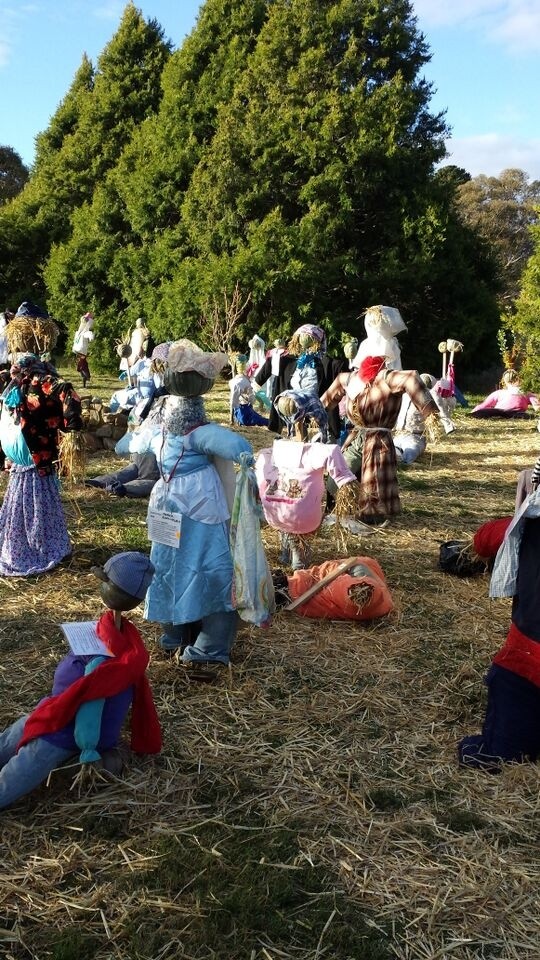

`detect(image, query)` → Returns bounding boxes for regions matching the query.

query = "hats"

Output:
[104,551,156,602]
[297,324,324,342]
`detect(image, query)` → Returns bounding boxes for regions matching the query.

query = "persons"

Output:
[0,552,162,810]
[465,369,540,418]
[0,305,469,683]
[447,456,540,775]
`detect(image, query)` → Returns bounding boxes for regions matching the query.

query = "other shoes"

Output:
[159,633,188,649]
[187,667,217,682]
[459,735,535,774]
